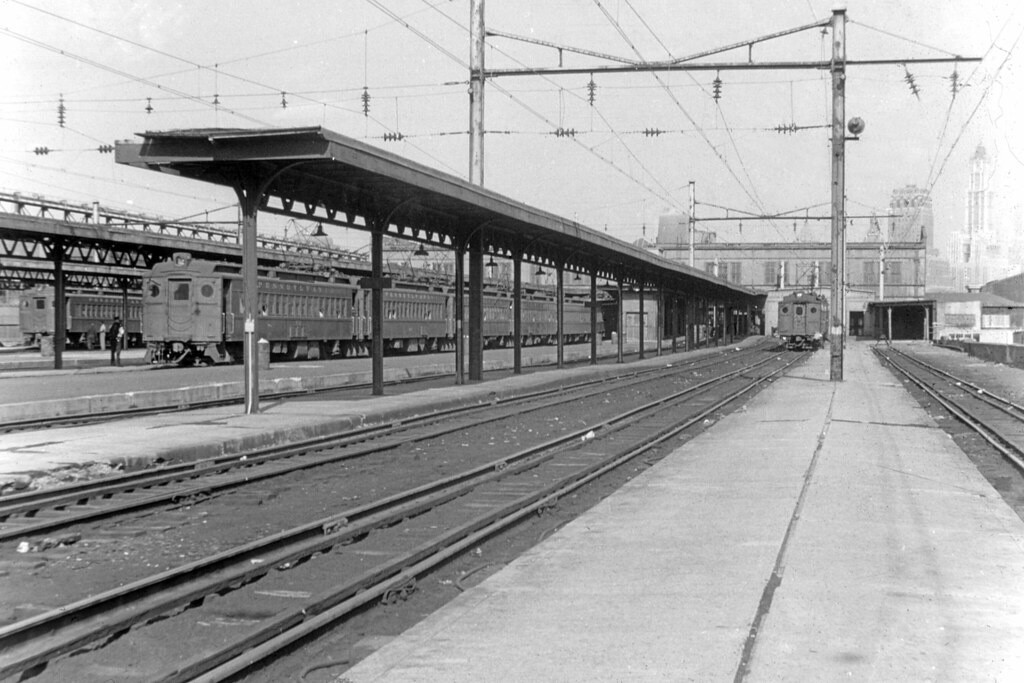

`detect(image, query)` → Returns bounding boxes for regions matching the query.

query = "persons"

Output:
[97,321,107,350]
[107,317,125,366]
[87,322,98,350]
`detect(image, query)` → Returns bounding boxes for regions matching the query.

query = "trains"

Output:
[778,292,827,352]
[142,251,606,368]
[19,285,140,350]
